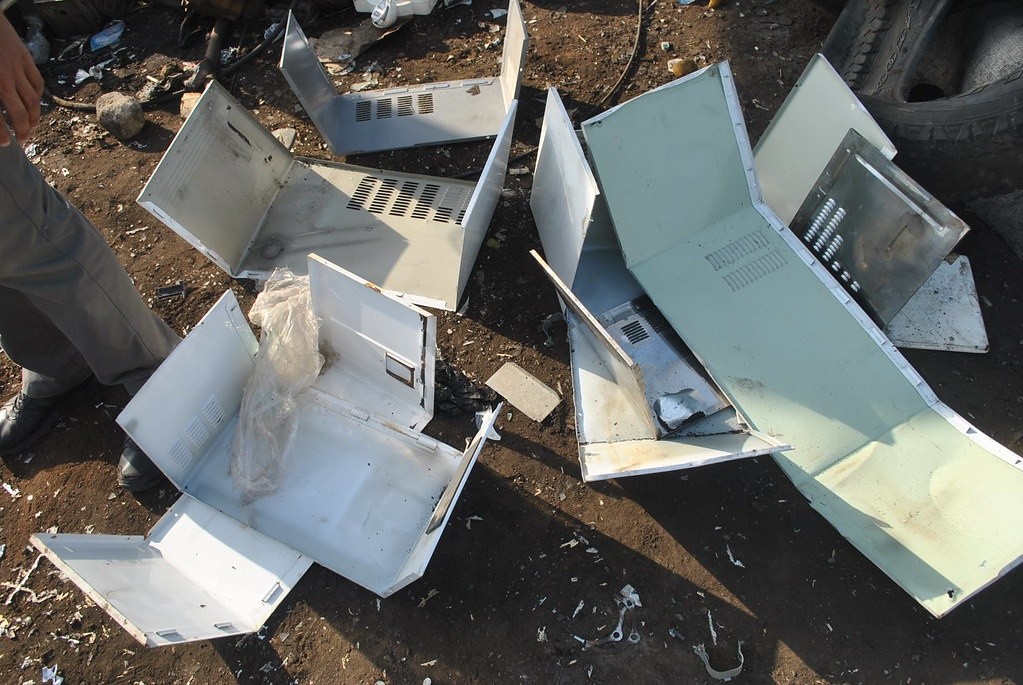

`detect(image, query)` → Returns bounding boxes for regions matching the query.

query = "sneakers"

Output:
[115,434,164,491]
[0,373,96,458]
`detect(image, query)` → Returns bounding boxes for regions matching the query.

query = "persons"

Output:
[0,8,182,490]
